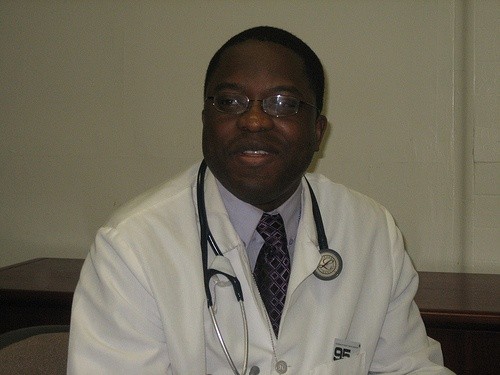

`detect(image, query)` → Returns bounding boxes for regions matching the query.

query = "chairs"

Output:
[0,325,70,375]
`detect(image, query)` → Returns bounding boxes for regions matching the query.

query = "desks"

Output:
[0,257,500,375]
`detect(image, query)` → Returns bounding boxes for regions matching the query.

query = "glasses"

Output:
[203,92,321,118]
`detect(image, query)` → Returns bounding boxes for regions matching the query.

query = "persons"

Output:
[66,26,457,375]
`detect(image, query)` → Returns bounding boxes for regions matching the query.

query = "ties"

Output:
[252,213,291,340]
